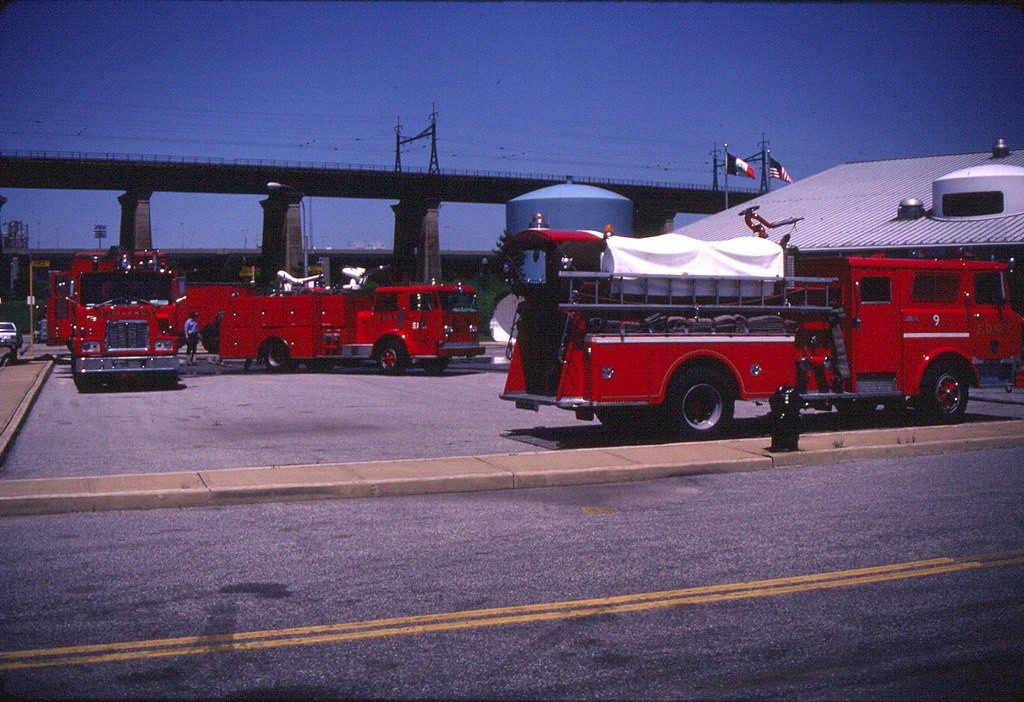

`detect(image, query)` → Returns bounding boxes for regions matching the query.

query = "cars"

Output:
[0,322,22,347]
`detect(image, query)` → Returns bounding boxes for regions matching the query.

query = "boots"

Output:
[186,354,194,366]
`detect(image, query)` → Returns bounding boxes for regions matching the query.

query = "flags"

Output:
[727,153,755,179]
[769,158,793,183]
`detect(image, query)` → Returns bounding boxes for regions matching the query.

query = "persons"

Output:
[185,313,198,365]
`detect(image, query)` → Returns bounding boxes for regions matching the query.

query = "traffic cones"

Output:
[770,378,807,454]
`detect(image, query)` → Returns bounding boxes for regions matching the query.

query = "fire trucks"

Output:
[489,204,1024,441]
[218,270,487,377]
[47,244,188,393]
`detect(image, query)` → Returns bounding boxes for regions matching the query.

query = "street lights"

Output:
[93,223,107,248]
[265,181,310,289]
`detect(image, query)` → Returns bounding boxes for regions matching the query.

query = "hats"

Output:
[189,311,197,318]
